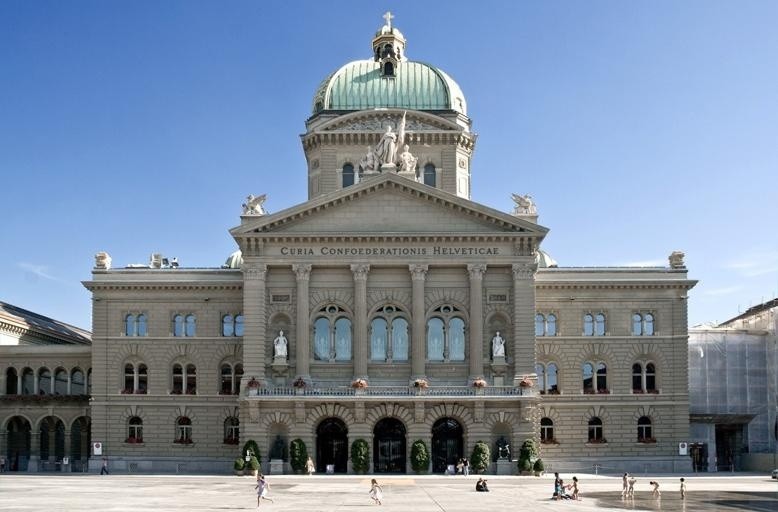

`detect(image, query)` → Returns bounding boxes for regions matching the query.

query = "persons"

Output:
[727,447,735,473]
[680,478,686,500]
[628,477,636,495]
[621,472,628,494]
[476,478,490,491]
[359,126,419,172]
[255,472,274,508]
[0,457,7,473]
[369,479,382,505]
[650,481,661,495]
[304,457,316,475]
[274,330,288,356]
[492,332,506,356]
[100,458,108,475]
[456,456,469,476]
[552,472,579,500]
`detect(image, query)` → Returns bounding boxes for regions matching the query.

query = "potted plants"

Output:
[234,456,261,475]
[533,459,545,477]
[291,438,307,474]
[410,439,429,475]
[519,459,531,476]
[351,438,370,474]
[471,439,490,473]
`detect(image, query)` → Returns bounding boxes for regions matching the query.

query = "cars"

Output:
[772,468,778,479]
